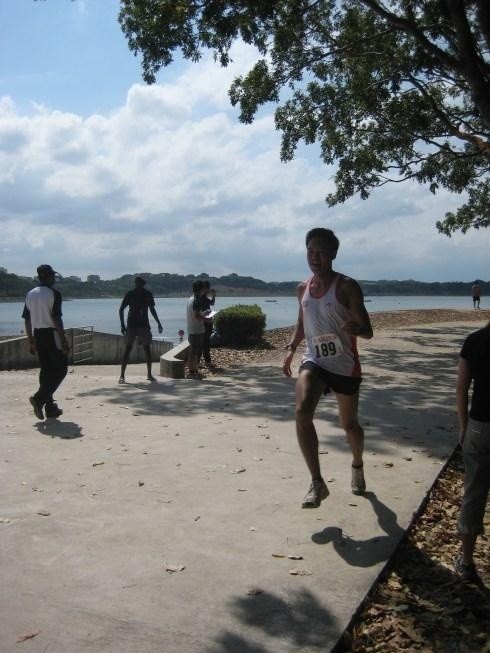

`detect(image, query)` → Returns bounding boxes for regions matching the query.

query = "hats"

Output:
[37,265,57,276]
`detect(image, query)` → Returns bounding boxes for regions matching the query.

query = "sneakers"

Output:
[147,375,155,381]
[204,363,216,368]
[119,376,125,384]
[352,463,366,495]
[30,397,44,420]
[45,404,62,417]
[302,479,329,508]
[188,370,206,379]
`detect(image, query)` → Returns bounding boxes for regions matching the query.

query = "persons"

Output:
[117,276,165,383]
[196,280,216,369]
[186,278,213,380]
[471,280,481,309]
[22,263,70,421]
[455,318,489,586]
[283,226,374,509]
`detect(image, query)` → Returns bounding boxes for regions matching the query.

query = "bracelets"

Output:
[285,343,297,354]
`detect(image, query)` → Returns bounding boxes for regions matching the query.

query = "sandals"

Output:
[455,555,474,579]
